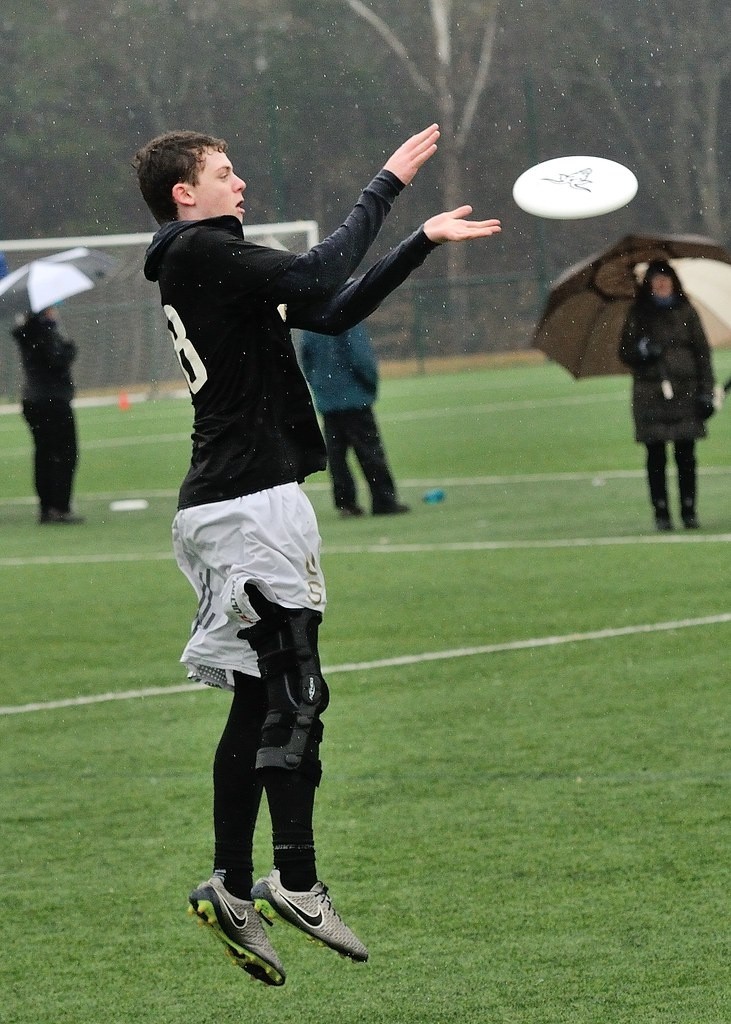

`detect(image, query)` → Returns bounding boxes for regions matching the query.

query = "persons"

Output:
[617,257,716,531]
[9,303,86,525]
[135,122,503,987]
[298,323,411,518]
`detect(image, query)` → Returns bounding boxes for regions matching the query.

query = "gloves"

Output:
[646,339,665,356]
[699,400,713,418]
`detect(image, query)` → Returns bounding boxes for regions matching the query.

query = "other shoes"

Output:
[340,504,364,516]
[681,512,699,528]
[371,500,409,514]
[655,513,673,532]
[39,509,82,523]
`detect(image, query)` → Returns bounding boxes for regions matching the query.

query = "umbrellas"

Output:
[0,246,118,319]
[530,232,731,382]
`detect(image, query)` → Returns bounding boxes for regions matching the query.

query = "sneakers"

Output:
[250,869,368,964]
[189,869,286,986]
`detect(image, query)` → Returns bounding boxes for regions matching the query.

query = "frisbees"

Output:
[512,156,639,221]
